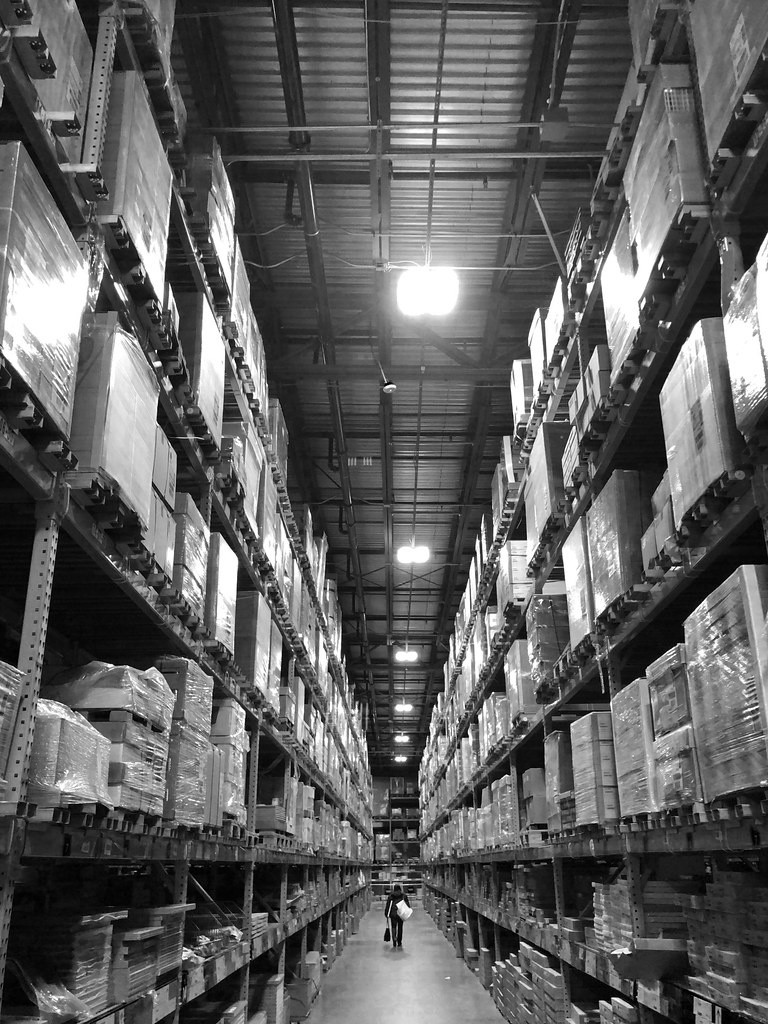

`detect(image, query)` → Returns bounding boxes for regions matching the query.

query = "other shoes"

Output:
[398,941,402,946]
[393,938,397,948]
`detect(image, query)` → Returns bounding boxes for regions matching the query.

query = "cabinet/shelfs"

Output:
[0,1,768,1023]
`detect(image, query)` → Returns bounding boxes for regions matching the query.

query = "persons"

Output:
[385,884,410,947]
[372,860,383,879]
[408,881,414,893]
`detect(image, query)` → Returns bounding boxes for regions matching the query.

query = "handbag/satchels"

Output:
[384,917,391,942]
[396,899,413,921]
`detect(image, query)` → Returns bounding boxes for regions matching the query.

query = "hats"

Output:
[394,885,401,891]
